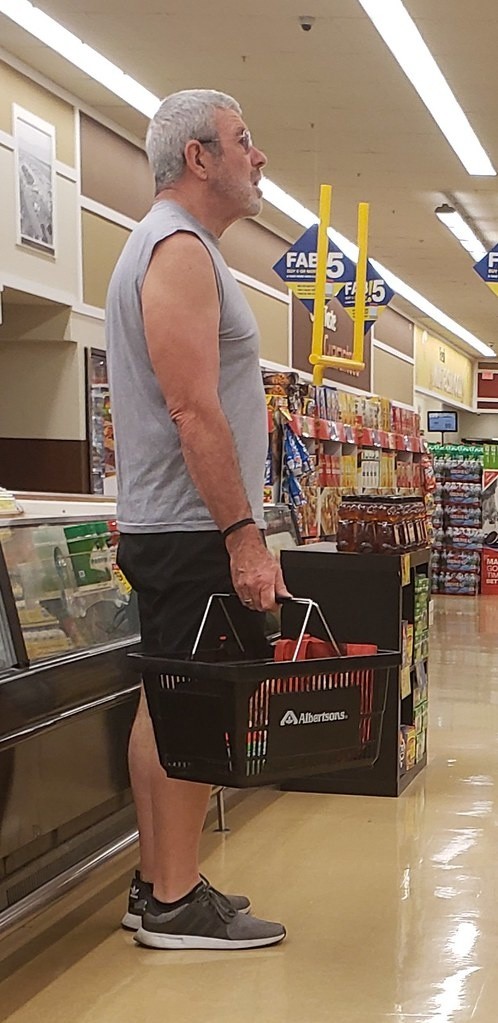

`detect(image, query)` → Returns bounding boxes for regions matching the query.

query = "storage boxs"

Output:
[263,371,415,538]
[483,445,498,470]
[278,541,430,800]
[480,548,498,595]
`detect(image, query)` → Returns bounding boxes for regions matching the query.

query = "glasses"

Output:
[198,129,253,153]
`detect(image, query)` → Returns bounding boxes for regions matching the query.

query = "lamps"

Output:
[434,204,488,262]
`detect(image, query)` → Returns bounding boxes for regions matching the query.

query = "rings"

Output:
[244,598,252,603]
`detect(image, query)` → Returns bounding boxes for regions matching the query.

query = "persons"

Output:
[105,89,293,949]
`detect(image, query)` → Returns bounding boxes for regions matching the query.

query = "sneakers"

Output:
[122,869,252,931]
[134,884,287,950]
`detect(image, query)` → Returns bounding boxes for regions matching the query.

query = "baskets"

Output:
[126,592,404,790]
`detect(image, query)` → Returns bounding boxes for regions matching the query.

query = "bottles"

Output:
[336,493,429,555]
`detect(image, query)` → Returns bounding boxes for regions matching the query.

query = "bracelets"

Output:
[220,517,255,540]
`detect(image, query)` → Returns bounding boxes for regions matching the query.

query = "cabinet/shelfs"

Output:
[268,412,427,546]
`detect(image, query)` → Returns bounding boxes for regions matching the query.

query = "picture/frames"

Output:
[11,102,57,258]
